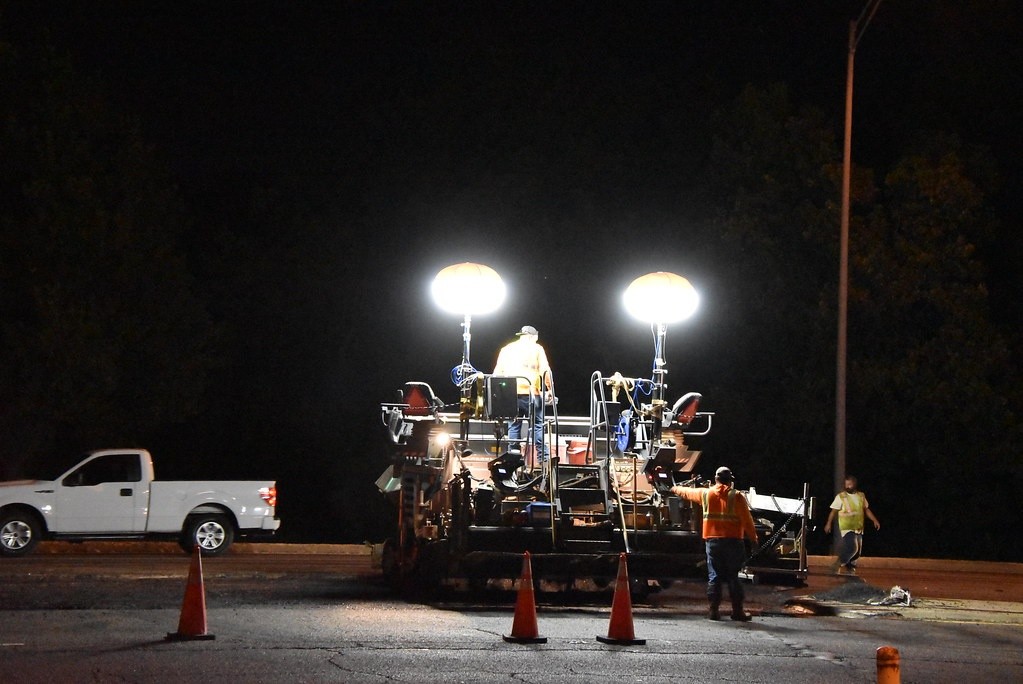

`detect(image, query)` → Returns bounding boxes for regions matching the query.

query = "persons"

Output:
[671,466,758,621]
[825,477,880,573]
[493,326,555,461]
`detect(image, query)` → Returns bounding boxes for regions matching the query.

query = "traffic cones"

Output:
[502,551,546,645]
[169,545,218,640]
[597,552,646,645]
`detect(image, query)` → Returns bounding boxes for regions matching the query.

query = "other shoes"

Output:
[710,612,721,620]
[831,563,841,576]
[731,612,752,621]
[846,567,856,574]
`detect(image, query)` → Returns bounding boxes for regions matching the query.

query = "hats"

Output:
[716,466,735,483]
[516,325,538,336]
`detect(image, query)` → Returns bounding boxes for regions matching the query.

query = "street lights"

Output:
[837,6,876,581]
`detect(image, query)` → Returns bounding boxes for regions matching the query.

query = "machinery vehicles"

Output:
[379,258,815,595]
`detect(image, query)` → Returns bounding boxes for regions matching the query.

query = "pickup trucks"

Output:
[3,447,279,552]
